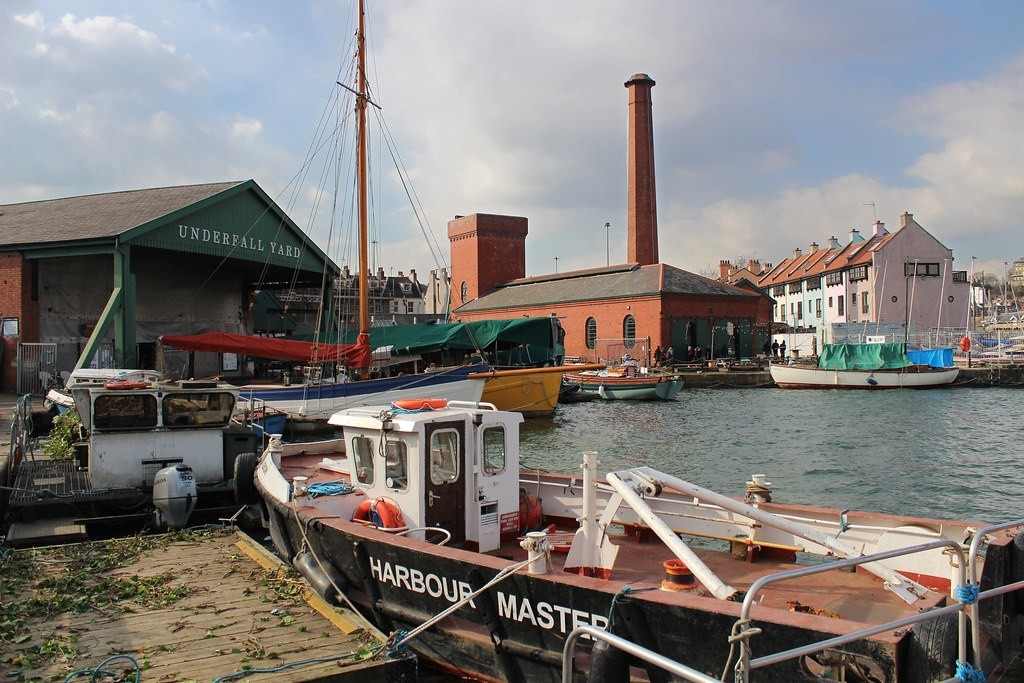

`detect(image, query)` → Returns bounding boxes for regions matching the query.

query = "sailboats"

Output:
[164,0,607,433]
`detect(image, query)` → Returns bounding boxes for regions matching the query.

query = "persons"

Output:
[654,346,675,367]
[763,339,786,359]
[688,343,729,362]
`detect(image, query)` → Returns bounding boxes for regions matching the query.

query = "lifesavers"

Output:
[959,337,971,351]
[391,398,447,409]
[104,381,148,390]
[350,496,405,537]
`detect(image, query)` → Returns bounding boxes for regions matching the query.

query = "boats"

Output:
[52,369,289,537]
[768,358,961,392]
[230,393,1023,683]
[277,314,567,424]
[558,381,604,406]
[562,357,687,401]
